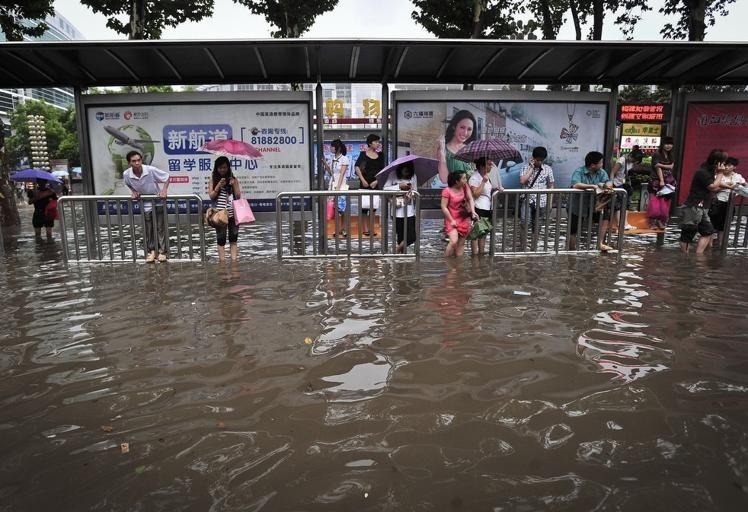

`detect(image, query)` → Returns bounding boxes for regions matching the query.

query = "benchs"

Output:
[607,208,668,235]
[323,213,382,240]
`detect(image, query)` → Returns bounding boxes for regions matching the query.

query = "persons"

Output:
[384,161,418,253]
[209,156,240,261]
[123,151,170,261]
[520,147,554,252]
[61,177,70,196]
[354,134,384,237]
[468,157,504,254]
[566,151,614,252]
[436,111,478,185]
[322,139,351,236]
[611,145,643,230]
[680,149,732,256]
[646,136,675,229]
[26,178,58,239]
[441,170,479,257]
[708,156,748,248]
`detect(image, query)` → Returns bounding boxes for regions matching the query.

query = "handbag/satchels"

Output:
[326,182,350,221]
[232,199,256,226]
[358,189,380,209]
[615,184,633,201]
[512,198,525,218]
[205,208,229,228]
[458,196,472,219]
[648,174,675,191]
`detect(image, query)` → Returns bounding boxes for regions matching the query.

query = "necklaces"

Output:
[559,103,581,143]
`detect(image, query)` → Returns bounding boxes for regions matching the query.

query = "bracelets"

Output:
[214,189,219,193]
[323,162,327,165]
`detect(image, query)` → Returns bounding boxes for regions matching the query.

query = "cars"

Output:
[431,152,529,189]
[72,166,81,177]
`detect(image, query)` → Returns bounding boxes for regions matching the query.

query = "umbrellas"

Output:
[595,181,611,196]
[374,154,439,191]
[73,168,82,173]
[10,168,62,192]
[736,183,747,199]
[453,139,518,175]
[195,137,262,179]
[465,214,495,241]
[50,171,70,179]
[656,184,676,199]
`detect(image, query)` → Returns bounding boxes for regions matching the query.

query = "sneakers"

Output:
[363,232,377,236]
[158,254,167,263]
[146,250,155,264]
[596,244,613,250]
[624,224,637,230]
[333,229,347,237]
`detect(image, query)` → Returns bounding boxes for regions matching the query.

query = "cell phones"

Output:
[407,183,411,185]
[221,178,225,180]
[530,156,533,161]
[716,160,722,165]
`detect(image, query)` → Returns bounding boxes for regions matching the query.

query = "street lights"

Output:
[26,113,50,171]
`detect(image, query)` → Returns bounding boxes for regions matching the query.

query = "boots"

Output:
[649,218,665,230]
